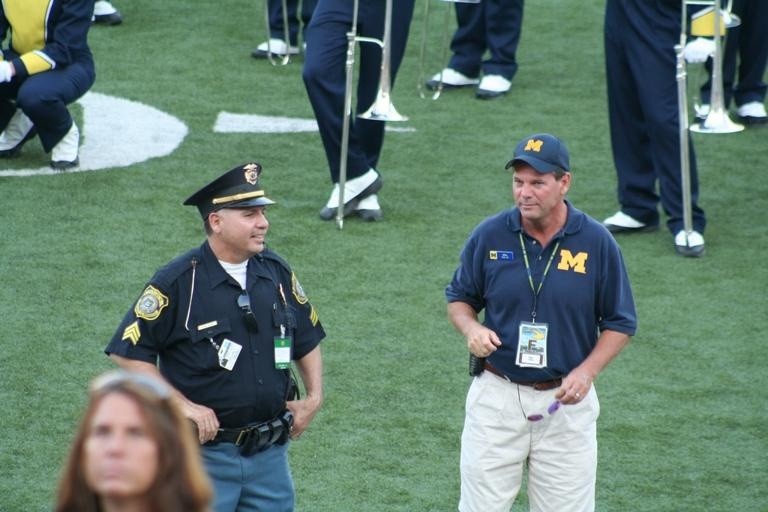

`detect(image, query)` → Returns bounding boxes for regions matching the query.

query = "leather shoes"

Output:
[474,75,512,101]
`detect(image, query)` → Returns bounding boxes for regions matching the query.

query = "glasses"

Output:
[519,395,561,422]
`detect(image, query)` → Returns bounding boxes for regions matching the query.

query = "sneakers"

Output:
[737,99,767,125]
[250,38,305,58]
[0,106,37,159]
[693,94,731,124]
[317,168,384,223]
[671,225,707,257]
[426,66,479,87]
[48,120,82,169]
[599,207,660,234]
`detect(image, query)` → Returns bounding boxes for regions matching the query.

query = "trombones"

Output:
[337,0,411,229]
[675,3,744,231]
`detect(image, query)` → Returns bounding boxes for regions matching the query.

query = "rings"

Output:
[575,392,580,397]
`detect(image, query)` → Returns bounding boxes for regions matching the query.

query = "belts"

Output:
[184,412,298,452]
[483,362,563,389]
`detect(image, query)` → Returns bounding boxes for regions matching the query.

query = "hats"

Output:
[504,132,572,176]
[182,161,275,212]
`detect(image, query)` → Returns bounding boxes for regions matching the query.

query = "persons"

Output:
[54,366,215,510]
[249,1,319,57]
[104,160,328,511]
[692,0,768,128]
[0,1,97,168]
[443,131,640,510]
[299,1,418,220]
[599,1,707,258]
[423,1,526,99]
